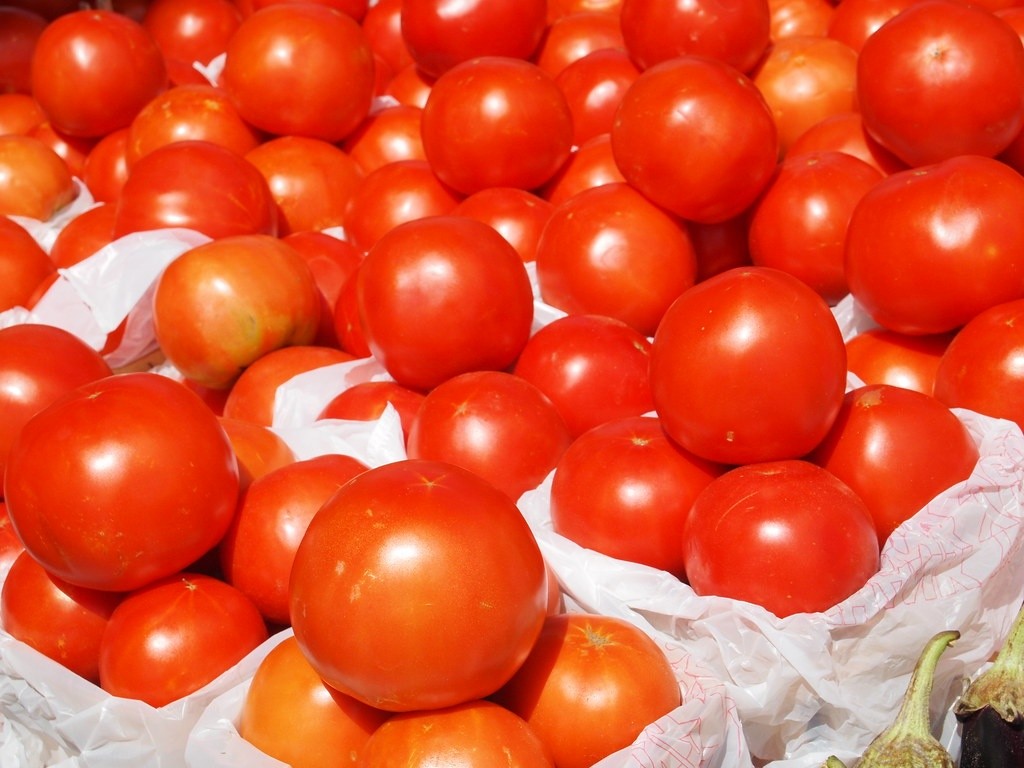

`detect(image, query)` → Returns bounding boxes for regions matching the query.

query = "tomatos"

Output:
[1,0,1024,767]
